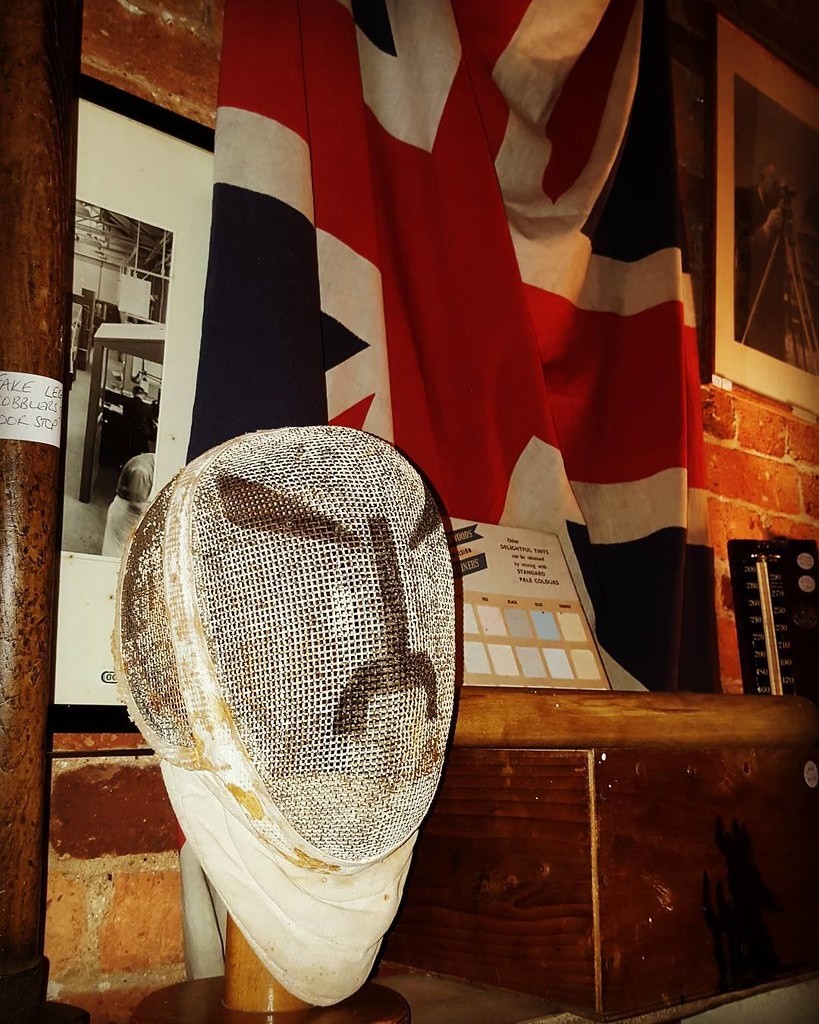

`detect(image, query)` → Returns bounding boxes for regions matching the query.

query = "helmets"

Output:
[114,425,459,1007]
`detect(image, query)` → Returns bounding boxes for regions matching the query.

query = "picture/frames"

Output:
[56,72,216,731]
[702,11,819,428]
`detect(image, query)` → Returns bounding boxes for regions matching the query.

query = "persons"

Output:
[103,385,156,558]
[735,158,788,361]
